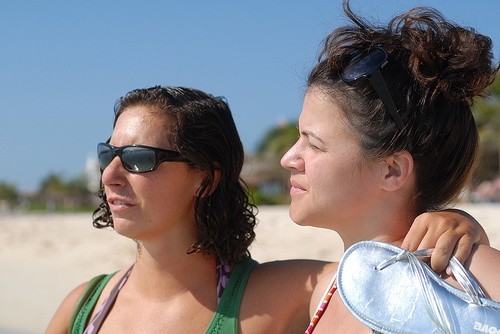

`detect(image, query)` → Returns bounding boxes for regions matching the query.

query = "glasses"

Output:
[96,143,190,173]
[333,46,403,129]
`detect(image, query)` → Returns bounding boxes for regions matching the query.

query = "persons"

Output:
[280,0,500,334]
[43,85,491,334]
